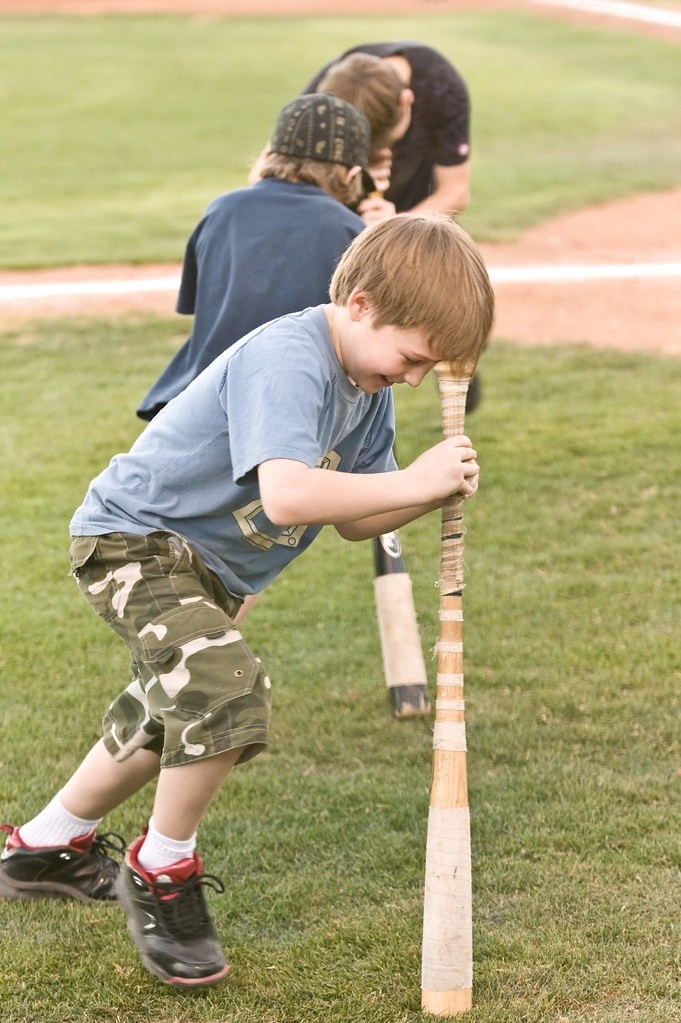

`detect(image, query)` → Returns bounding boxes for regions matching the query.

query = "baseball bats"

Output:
[418,357,479,1019]
[358,145,432,717]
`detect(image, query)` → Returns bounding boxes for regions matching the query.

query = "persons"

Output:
[136,93,379,421]
[1,211,495,991]
[305,38,485,413]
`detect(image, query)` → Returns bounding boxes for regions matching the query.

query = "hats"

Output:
[270,92,377,192]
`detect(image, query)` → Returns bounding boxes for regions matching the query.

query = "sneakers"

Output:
[0,825,123,903]
[114,834,230,986]
